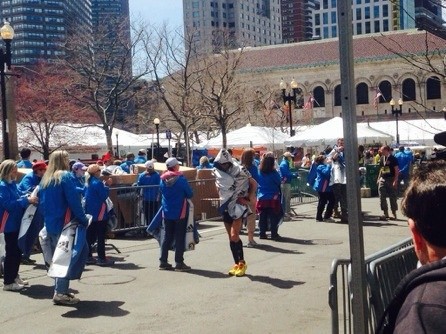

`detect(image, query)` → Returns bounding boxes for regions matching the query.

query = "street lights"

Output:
[389,97,404,143]
[281,80,299,137]
[0,20,21,160]
[153,117,161,148]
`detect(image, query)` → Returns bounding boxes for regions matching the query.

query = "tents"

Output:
[194,124,289,153]
[283,117,393,152]
[369,118,446,147]
[0,121,148,159]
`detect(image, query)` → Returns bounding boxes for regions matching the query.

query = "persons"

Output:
[195,157,214,169]
[256,150,297,240]
[0,159,38,291]
[19,163,47,265]
[133,157,193,270]
[108,149,147,174]
[302,139,347,223]
[17,148,33,168]
[377,160,446,334]
[358,144,426,220]
[70,150,114,266]
[32,159,37,166]
[211,148,258,278]
[39,151,89,303]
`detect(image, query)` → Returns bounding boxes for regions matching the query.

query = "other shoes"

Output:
[317,210,349,223]
[377,215,390,220]
[270,235,283,240]
[247,240,257,248]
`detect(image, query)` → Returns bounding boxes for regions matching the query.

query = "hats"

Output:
[145,161,154,170]
[283,151,295,157]
[32,162,47,171]
[165,157,183,168]
[138,149,148,155]
[72,163,87,171]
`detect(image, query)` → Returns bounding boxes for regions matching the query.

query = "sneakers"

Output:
[158,263,172,269]
[53,289,80,304]
[15,276,29,285]
[229,262,247,277]
[20,257,37,265]
[3,281,24,291]
[97,256,115,265]
[85,256,97,264]
[174,263,192,271]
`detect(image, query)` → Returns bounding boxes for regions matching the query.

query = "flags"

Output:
[373,88,381,110]
[305,97,316,109]
